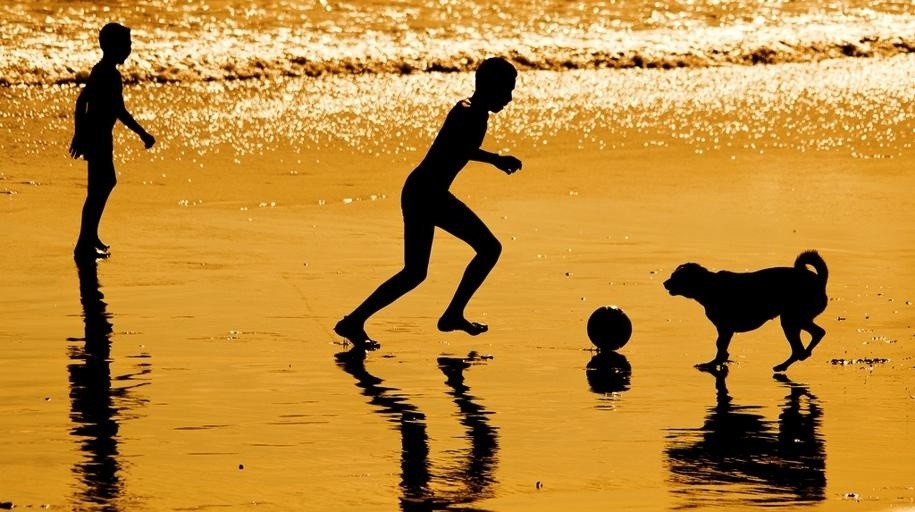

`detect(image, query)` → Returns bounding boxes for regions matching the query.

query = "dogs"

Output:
[662,249,829,372]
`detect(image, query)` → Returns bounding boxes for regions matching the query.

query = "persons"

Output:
[60,266,155,509]
[334,347,505,511]
[68,22,158,266]
[331,53,525,353]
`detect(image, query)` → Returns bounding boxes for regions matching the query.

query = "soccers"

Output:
[587,305,631,350]
